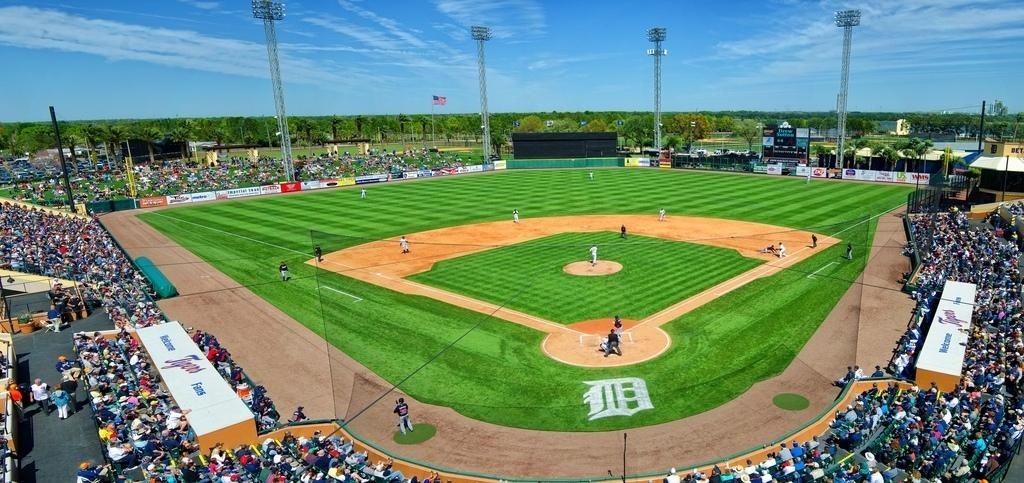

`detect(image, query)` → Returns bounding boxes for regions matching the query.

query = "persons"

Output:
[665,202,1023,482]
[1,145,472,206]
[589,243,598,265]
[659,207,666,220]
[600,336,609,351]
[1,196,465,482]
[612,315,622,336]
[621,224,626,236]
[512,207,520,222]
[603,327,623,357]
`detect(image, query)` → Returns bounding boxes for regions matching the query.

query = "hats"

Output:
[735,464,751,482]
[79,461,91,469]
[865,450,875,461]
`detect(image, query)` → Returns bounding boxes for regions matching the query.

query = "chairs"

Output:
[0,164,1024,483]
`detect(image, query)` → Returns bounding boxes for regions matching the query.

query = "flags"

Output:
[513,122,520,127]
[432,94,447,107]
[618,120,624,125]
[580,120,587,125]
[547,120,554,126]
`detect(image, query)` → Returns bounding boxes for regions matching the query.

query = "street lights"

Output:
[251,0,297,182]
[471,25,492,164]
[646,26,667,151]
[832,7,861,169]
